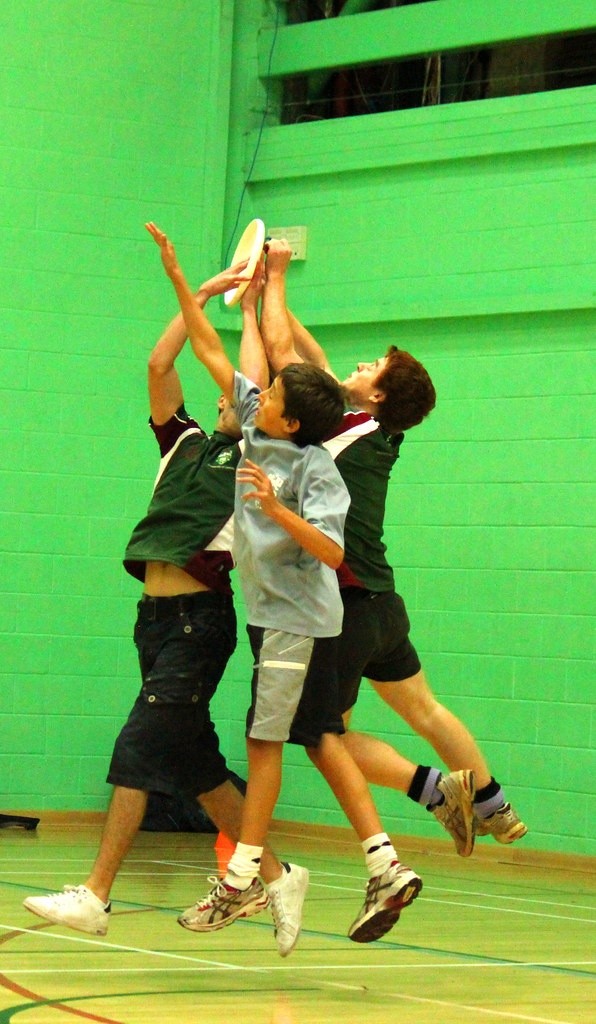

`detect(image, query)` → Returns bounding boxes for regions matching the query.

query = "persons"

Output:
[25,218,528,958]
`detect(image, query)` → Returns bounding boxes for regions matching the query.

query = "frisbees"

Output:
[224,217,266,307]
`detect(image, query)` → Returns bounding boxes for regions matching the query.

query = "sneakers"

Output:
[23,884,111,936]
[266,861,309,957]
[471,802,528,844]
[348,861,422,943]
[177,877,271,932]
[428,769,476,857]
